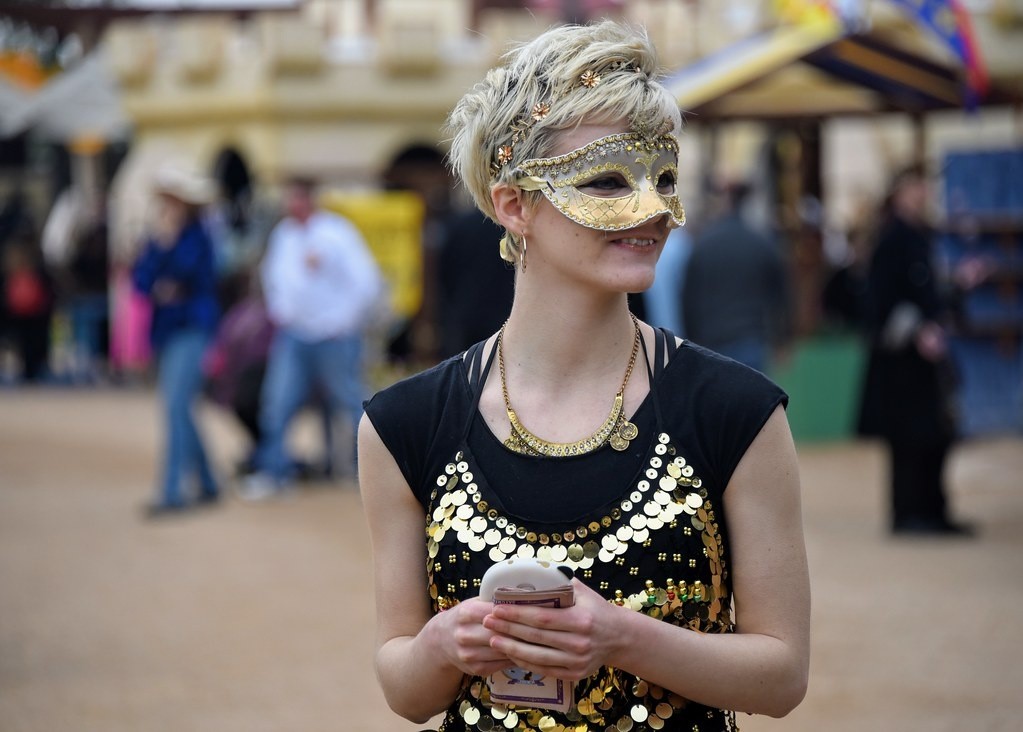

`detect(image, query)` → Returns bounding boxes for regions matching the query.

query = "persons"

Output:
[128,169,227,514]
[352,24,816,732]
[850,165,990,542]
[243,161,390,502]
[376,139,801,412]
[0,148,123,398]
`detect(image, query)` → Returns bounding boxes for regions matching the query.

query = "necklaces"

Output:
[494,312,645,460]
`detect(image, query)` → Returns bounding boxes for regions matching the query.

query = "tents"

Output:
[651,11,1022,339]
